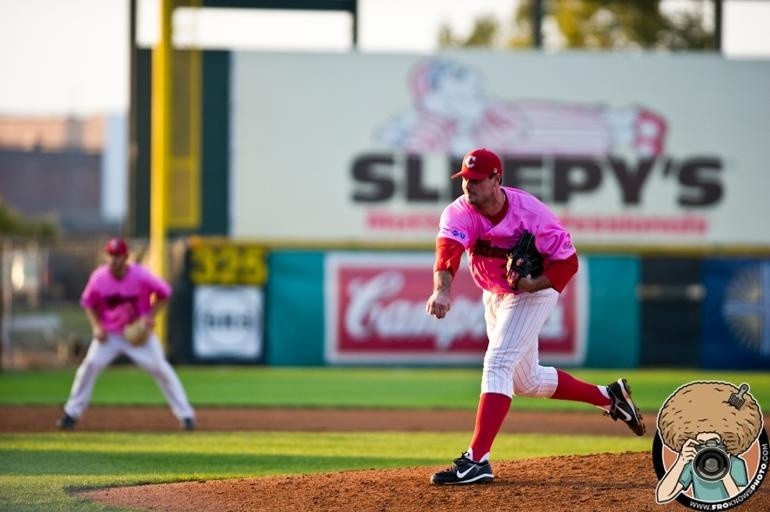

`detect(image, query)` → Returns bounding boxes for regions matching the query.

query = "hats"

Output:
[451,149,503,181]
[104,237,128,256]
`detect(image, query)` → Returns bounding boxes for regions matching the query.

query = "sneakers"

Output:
[603,378,646,436]
[430,453,494,485]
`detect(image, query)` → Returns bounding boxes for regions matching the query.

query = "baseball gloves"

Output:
[123,316,154,347]
[506,233,544,291]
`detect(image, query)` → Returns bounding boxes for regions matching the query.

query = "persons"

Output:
[426,148,646,485]
[57,238,196,430]
